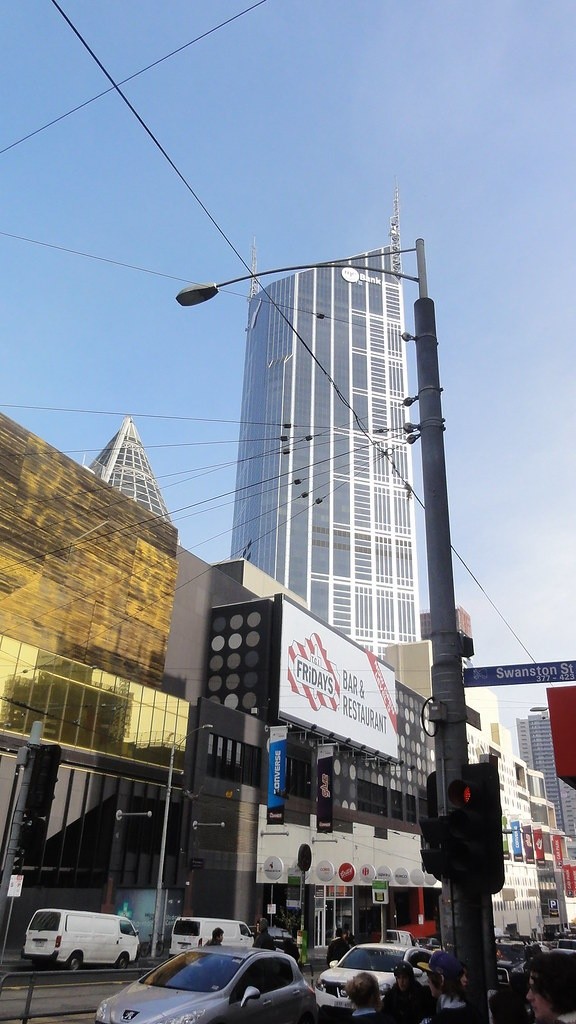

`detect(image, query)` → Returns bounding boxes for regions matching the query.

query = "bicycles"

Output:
[140,933,164,957]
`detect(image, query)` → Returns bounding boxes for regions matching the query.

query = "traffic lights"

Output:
[416,757,505,899]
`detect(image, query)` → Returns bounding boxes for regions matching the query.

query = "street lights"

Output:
[148,724,212,960]
[177,236,488,1024]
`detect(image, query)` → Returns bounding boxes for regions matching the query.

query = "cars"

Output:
[93,945,322,1024]
[314,944,432,1020]
[417,938,441,950]
[493,926,576,990]
[267,925,294,949]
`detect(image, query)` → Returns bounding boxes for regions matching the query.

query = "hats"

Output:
[428,951,465,980]
[394,961,413,976]
[523,953,576,1012]
[257,918,268,931]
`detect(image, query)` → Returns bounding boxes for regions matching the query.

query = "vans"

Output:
[19,909,140,971]
[384,928,419,950]
[170,918,255,960]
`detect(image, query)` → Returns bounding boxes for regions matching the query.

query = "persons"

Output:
[368,930,380,943]
[280,938,300,961]
[327,928,355,968]
[488,950,576,1024]
[198,928,224,958]
[254,918,274,950]
[344,973,389,1024]
[382,951,484,1024]
